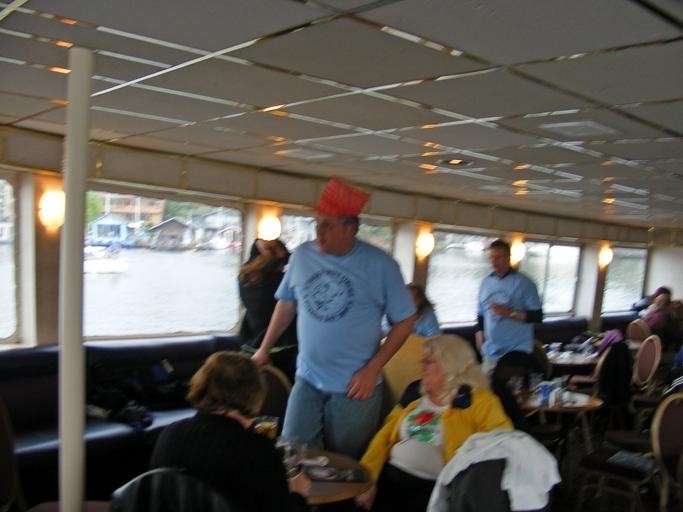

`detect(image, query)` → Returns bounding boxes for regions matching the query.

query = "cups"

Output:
[253,415,279,443]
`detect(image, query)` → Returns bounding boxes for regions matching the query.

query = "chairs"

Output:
[534,319,682,511]
[441,453,555,509]
[107,467,233,511]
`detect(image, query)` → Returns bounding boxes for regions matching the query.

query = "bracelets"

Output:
[509,310,517,319]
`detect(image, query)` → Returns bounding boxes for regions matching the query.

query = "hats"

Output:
[314,177,371,220]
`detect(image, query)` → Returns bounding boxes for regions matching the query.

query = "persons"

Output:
[251,177,418,463]
[380,281,440,337]
[148,350,311,512]
[351,333,515,512]
[235,237,292,350]
[473,238,543,428]
[636,286,683,376]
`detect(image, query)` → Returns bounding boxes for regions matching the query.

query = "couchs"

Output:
[0,336,240,512]
